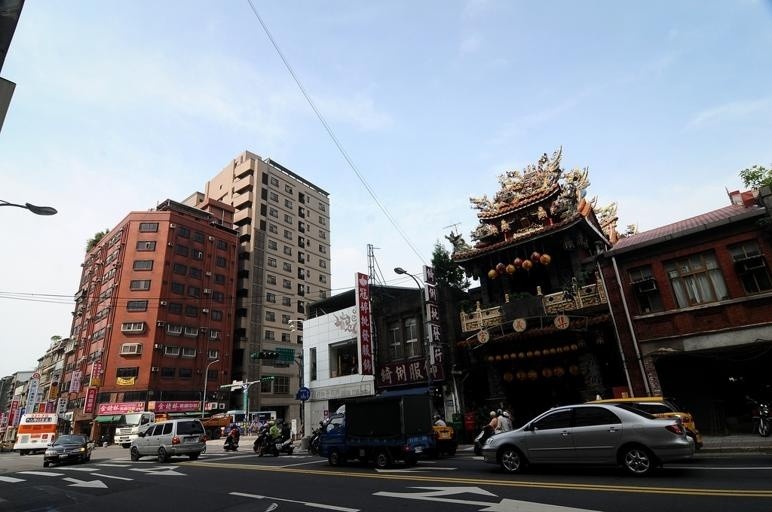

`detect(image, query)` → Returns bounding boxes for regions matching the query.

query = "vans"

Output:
[129,417,208,463]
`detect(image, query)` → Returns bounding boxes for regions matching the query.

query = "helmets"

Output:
[268,421,276,426]
[490,411,497,418]
[434,414,440,420]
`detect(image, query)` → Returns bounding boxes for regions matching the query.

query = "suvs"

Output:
[583,395,702,451]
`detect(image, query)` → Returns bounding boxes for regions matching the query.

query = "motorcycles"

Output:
[741,394,772,437]
[281,438,295,454]
[473,426,493,455]
[254,426,283,456]
[309,427,321,454]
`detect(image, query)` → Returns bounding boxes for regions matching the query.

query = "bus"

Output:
[13,412,73,456]
[224,410,277,435]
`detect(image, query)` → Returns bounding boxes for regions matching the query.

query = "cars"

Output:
[481,403,696,478]
[44,434,95,468]
[0,439,15,452]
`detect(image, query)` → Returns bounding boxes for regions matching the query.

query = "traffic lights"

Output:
[262,351,281,360]
[264,377,272,383]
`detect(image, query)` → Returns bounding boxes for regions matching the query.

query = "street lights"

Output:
[0,200,58,216]
[202,359,221,419]
[394,266,436,427]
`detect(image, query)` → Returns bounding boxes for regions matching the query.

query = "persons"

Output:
[433,414,446,425]
[484,404,514,433]
[225,418,290,453]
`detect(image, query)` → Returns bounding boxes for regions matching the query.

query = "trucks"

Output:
[153,413,233,440]
[430,416,458,457]
[112,410,158,449]
[318,386,437,470]
